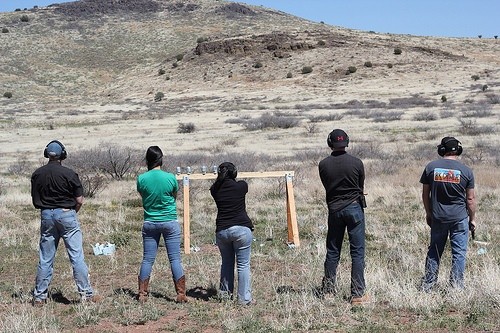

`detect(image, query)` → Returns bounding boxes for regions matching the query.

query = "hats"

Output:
[330,129,349,148]
[217,162,237,179]
[442,137,458,151]
[46,142,62,157]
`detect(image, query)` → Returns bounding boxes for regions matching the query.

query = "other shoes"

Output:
[80,295,103,303]
[352,297,362,306]
[33,301,43,307]
[322,291,333,299]
[246,299,257,306]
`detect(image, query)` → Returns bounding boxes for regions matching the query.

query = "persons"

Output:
[31,140,98,307]
[317,129,372,302]
[137,147,188,303]
[419,138,476,288]
[210,162,254,305]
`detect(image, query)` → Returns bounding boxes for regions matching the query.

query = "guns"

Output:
[469,225,475,240]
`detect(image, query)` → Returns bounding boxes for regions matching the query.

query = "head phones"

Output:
[327,131,349,147]
[217,164,237,179]
[437,139,462,156]
[44,140,67,160]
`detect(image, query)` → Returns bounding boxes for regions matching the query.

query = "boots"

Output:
[138,275,150,302]
[173,275,192,303]
